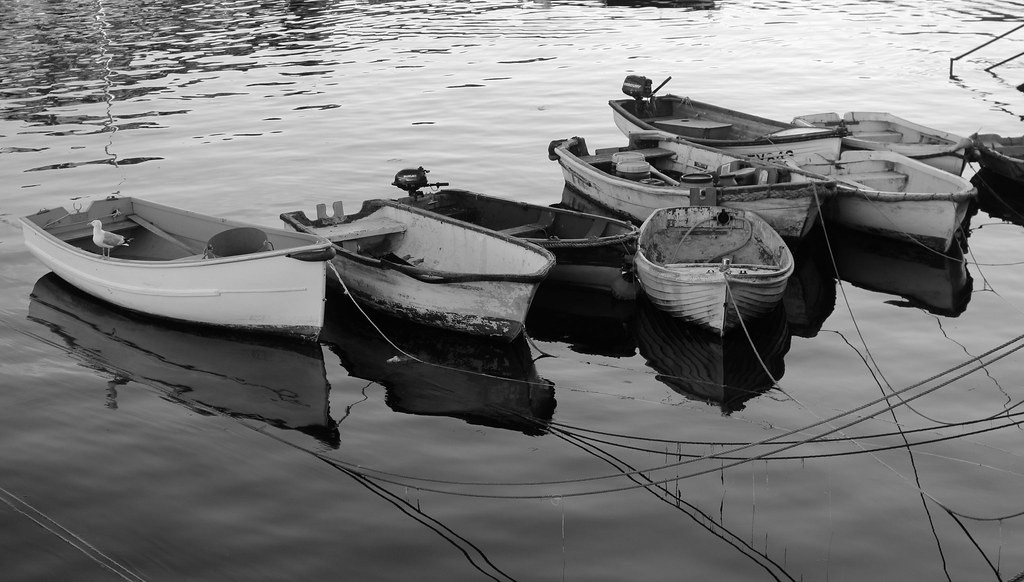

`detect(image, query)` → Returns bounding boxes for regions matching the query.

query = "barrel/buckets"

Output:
[616,161,651,181]
[207,227,267,259]
[681,173,714,190]
[717,174,737,186]
[611,152,645,176]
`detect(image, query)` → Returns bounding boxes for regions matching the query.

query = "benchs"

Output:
[578,147,676,165]
[431,202,482,222]
[308,216,406,242]
[839,167,913,193]
[642,115,680,126]
[991,145,1024,157]
[53,219,154,243]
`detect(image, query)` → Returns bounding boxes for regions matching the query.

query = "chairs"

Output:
[855,129,904,142]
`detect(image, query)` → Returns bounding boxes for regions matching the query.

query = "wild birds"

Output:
[88,220,131,261]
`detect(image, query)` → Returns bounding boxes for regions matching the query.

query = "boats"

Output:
[388,168,640,297]
[279,199,559,347]
[17,195,327,347]
[966,129,1020,185]
[630,202,798,335]
[548,128,836,246]
[798,148,975,250]
[789,111,974,178]
[607,75,843,175]
